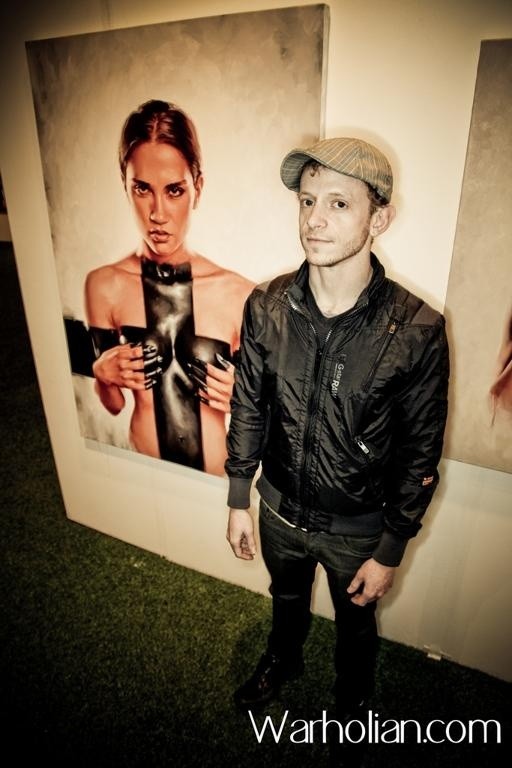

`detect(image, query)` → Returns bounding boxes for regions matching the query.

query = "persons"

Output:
[82,100,256,477]
[226,139,450,725]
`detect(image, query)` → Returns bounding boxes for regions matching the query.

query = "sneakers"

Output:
[233,656,307,716]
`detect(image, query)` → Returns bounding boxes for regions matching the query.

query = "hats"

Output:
[281,137,394,202]
